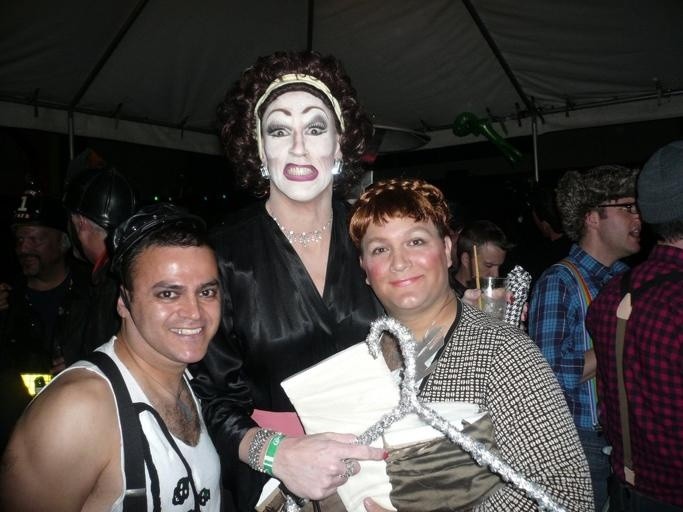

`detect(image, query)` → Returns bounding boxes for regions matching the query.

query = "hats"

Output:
[637,140,683,224]
[110,202,208,274]
[9,174,136,235]
[555,164,639,239]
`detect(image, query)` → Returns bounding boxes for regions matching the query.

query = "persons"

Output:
[445,143,683,511]
[277,179,595,511]
[0,208,246,512]
[185,44,388,512]
[1,144,166,437]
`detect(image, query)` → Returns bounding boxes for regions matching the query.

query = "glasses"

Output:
[583,201,641,218]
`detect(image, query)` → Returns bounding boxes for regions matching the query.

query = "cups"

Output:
[478,276,506,321]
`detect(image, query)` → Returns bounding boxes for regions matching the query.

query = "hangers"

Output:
[285,316,569,512]
